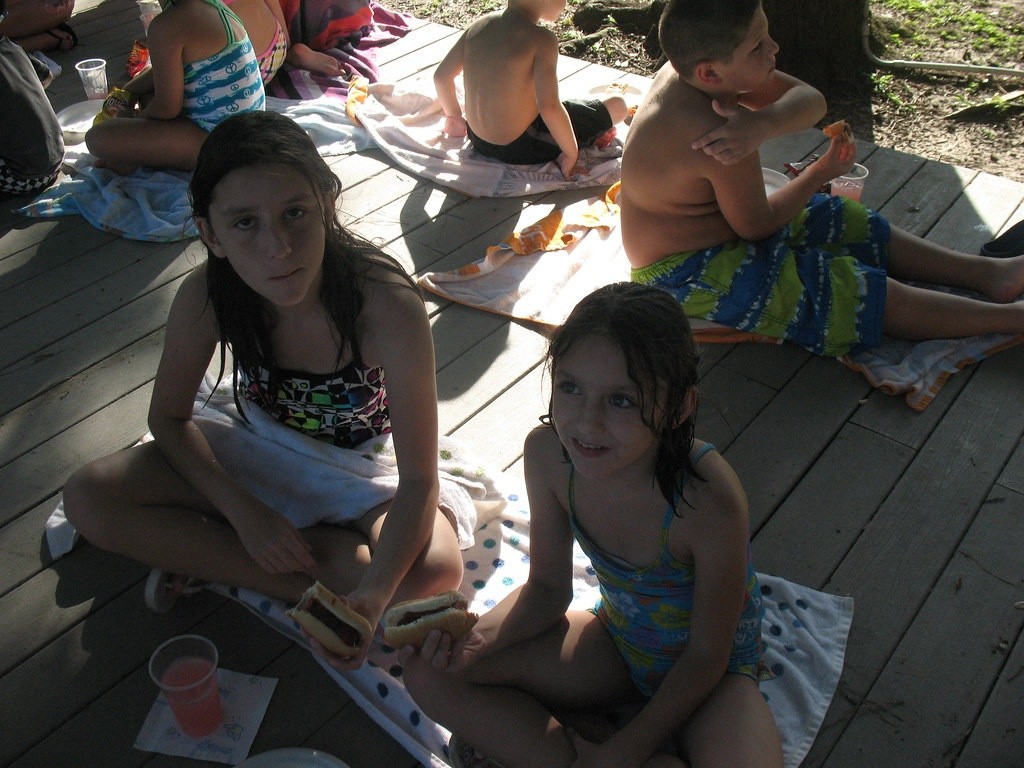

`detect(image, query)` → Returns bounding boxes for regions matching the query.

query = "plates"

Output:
[759,166,790,199]
[56,98,106,154]
[232,747,351,768]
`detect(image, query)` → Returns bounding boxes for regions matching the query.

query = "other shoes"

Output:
[449,734,504,768]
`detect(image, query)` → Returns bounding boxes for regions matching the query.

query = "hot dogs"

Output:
[382,590,479,650]
[285,581,372,657]
[824,120,855,143]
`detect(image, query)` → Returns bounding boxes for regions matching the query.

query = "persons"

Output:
[0,0,79,50]
[393,282,788,768]
[0,37,66,196]
[433,0,629,179]
[619,1,1024,357]
[221,0,345,91]
[84,0,265,175]
[59,110,466,672]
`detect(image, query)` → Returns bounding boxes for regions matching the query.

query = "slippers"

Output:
[48,22,78,52]
[145,567,210,612]
[981,220,1024,256]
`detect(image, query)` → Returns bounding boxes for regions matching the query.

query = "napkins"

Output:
[133,667,278,764]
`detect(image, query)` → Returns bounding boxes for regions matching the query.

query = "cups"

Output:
[831,163,870,203]
[148,633,224,739]
[75,58,108,100]
[140,12,160,37]
